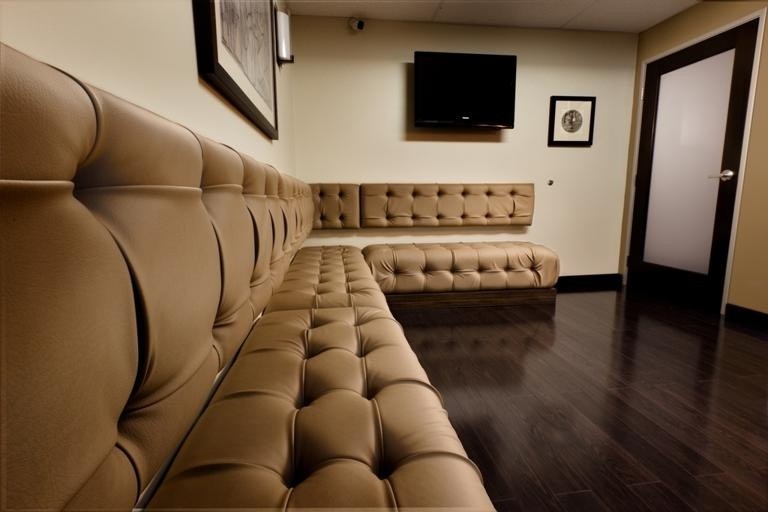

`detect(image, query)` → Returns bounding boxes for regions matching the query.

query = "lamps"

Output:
[275,6,295,66]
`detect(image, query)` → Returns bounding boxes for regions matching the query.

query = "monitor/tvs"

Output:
[413,51,517,129]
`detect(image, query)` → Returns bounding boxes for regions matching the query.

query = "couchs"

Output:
[0,39,557,512]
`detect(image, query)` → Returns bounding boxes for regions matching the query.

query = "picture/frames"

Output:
[192,0,279,140]
[547,95,596,148]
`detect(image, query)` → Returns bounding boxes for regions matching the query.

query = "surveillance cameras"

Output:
[352,20,364,31]
